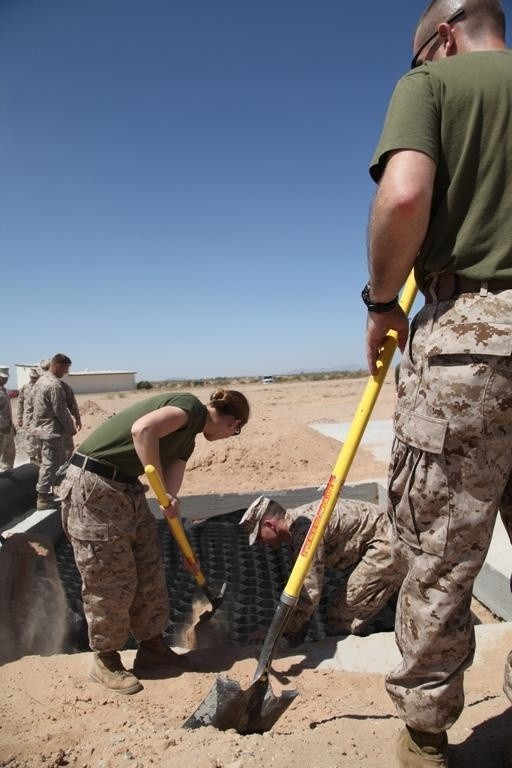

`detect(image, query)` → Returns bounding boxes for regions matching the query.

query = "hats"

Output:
[0,366,10,378]
[239,494,271,546]
[29,358,51,378]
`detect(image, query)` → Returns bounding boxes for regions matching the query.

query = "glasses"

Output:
[409,9,466,65]
[232,424,241,436]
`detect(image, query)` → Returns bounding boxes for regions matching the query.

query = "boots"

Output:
[36,493,62,510]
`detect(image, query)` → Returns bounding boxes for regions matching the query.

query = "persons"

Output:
[28,359,82,463]
[0,365,17,471]
[236,490,406,652]
[16,368,43,464]
[29,352,78,510]
[360,0,512,767]
[54,388,250,696]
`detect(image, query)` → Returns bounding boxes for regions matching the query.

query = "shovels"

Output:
[145,465,228,630]
[181,266,418,735]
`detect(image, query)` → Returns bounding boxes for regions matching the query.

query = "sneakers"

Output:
[396,728,450,768]
[92,655,143,696]
[134,640,191,670]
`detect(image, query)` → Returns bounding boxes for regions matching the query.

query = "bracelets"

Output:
[359,281,401,314]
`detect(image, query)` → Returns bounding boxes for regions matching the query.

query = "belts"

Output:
[423,271,512,303]
[71,452,138,484]
